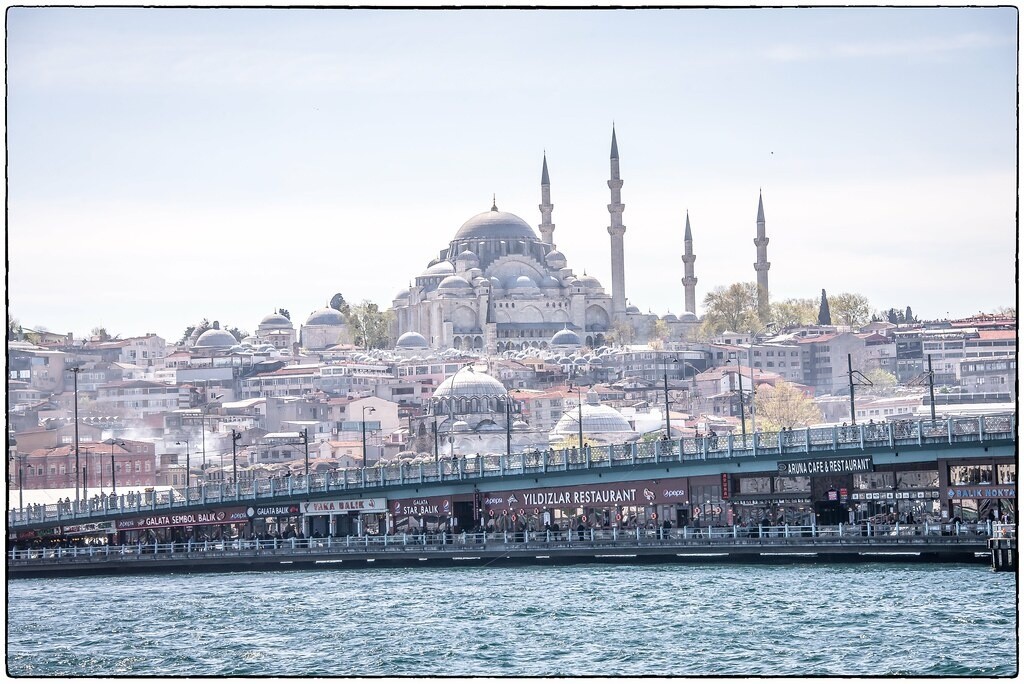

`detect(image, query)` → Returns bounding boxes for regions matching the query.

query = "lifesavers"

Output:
[694,507,701,514]
[582,515,587,523]
[511,515,516,522]
[616,514,622,521]
[534,508,539,515]
[520,508,524,515]
[502,509,508,516]
[651,513,657,519]
[715,506,722,514]
[489,509,494,516]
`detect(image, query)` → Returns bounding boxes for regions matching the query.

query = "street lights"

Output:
[663,356,679,448]
[298,428,308,474]
[232,428,242,484]
[566,384,584,463]
[422,403,439,477]
[19,455,31,520]
[176,439,189,486]
[362,405,376,466]
[78,446,95,498]
[202,393,224,483]
[507,388,521,473]
[449,361,473,461]
[66,365,85,513]
[726,354,748,450]
[750,321,776,429]
[112,440,125,493]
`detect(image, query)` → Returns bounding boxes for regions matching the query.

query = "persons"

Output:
[695,428,720,450]
[532,443,590,464]
[777,426,795,444]
[623,441,631,460]
[405,462,412,477]
[10,491,142,523]
[660,434,675,456]
[840,419,914,443]
[451,452,482,474]
[8,508,1012,559]
[268,467,382,490]
[753,427,762,446]
